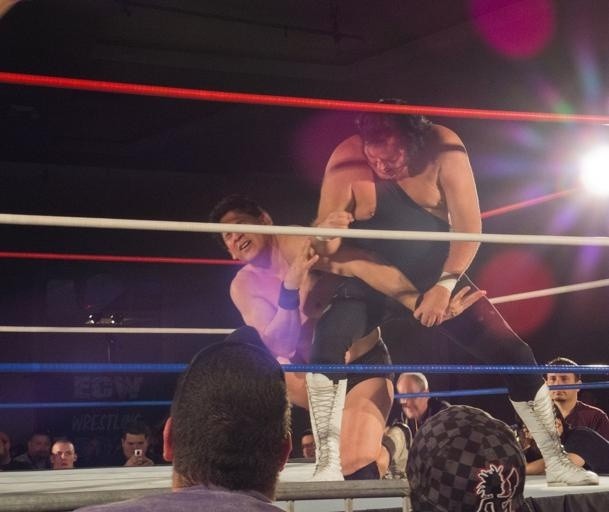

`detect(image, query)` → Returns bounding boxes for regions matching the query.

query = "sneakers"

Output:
[381,424,413,480]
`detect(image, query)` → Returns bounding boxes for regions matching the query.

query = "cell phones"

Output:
[135,449,143,457]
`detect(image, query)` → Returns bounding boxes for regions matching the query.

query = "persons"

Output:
[0,408,168,472]
[304,94,604,487]
[67,340,294,512]
[211,198,487,481]
[504,356,608,478]
[300,431,315,460]
[384,371,463,442]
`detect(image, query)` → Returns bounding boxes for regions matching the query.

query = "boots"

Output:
[509,379,600,487]
[304,373,347,481]
